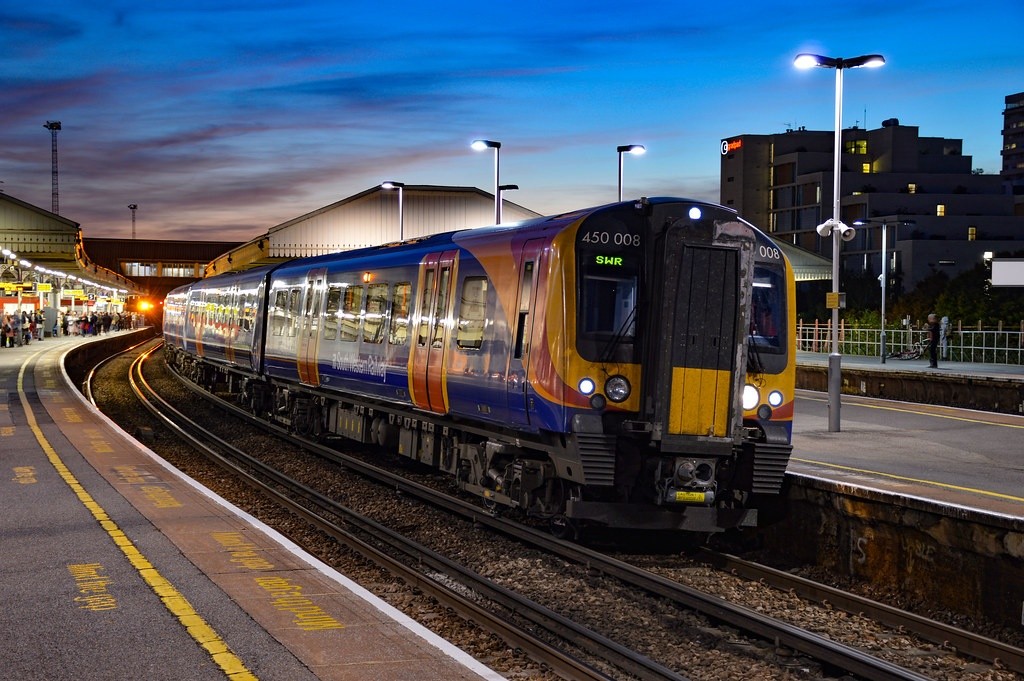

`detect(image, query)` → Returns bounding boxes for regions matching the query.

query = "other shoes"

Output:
[927,365,937,368]
[89,334,92,337]
[83,334,85,337]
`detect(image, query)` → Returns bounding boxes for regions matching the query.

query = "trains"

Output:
[163,197,797,561]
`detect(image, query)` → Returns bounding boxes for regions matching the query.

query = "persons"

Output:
[0,309,146,348]
[927,314,941,368]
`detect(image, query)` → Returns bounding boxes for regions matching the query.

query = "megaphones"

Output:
[816,219,834,237]
[839,222,855,241]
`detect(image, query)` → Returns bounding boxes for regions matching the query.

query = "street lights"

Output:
[616,144,648,201]
[854,218,911,363]
[382,180,405,241]
[791,52,887,433]
[471,138,502,224]
